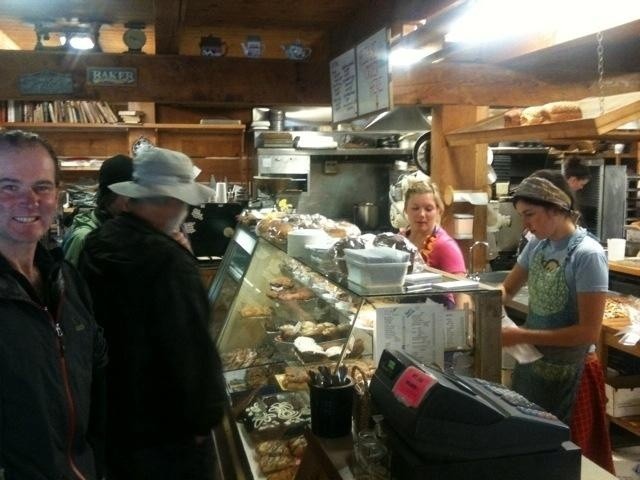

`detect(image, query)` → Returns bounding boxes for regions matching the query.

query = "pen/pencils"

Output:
[308,366,349,387]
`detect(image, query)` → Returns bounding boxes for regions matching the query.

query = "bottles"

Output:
[352,427,393,480]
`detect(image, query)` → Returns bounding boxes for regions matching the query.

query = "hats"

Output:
[107,145,216,206]
[96,155,132,207]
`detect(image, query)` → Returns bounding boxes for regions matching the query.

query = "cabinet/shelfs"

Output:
[0,97,256,213]
[603,293,640,437]
[206,223,501,479]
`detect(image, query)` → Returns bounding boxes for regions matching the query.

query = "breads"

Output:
[220,212,418,480]
[504,101,583,129]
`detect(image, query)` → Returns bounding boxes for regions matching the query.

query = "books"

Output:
[0,95,148,129]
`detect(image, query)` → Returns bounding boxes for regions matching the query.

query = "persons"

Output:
[0,130,109,479]
[75,144,233,480]
[392,180,474,349]
[562,158,600,238]
[492,166,617,476]
[58,155,132,266]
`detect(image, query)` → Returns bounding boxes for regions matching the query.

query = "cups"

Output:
[606,237,626,262]
[307,375,354,439]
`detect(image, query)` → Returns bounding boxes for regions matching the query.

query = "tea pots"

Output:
[240,36,266,59]
[280,39,312,60]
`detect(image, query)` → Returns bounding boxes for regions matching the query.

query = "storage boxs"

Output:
[606,377,640,418]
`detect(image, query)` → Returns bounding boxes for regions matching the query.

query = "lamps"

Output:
[60,31,95,50]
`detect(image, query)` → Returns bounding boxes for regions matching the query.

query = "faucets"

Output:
[470,241,490,277]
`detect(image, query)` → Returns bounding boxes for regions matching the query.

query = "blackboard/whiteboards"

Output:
[331,27,389,124]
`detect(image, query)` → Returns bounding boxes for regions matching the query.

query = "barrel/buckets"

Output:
[607,238,626,261]
[496,182,509,194]
